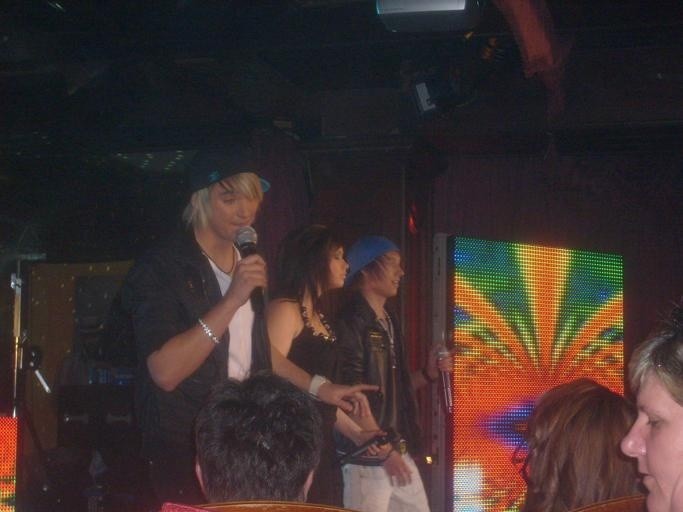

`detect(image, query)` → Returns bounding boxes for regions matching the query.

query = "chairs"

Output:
[572,492,648,512]
[194,500,357,512]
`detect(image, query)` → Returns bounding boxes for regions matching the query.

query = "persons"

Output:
[510,377,643,511]
[262,222,382,512]
[619,300,682,511]
[121,143,384,511]
[340,235,457,512]
[160,372,322,510]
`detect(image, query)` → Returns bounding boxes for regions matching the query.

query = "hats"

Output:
[190,136,271,196]
[339,234,401,286]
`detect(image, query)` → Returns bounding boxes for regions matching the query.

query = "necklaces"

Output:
[202,247,234,275]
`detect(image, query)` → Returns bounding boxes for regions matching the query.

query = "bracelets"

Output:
[197,317,223,344]
[421,366,432,384]
[381,447,394,462]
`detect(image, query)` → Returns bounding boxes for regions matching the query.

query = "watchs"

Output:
[308,373,331,400]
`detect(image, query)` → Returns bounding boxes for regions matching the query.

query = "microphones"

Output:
[233,225,266,315]
[328,434,390,470]
[438,346,454,415]
